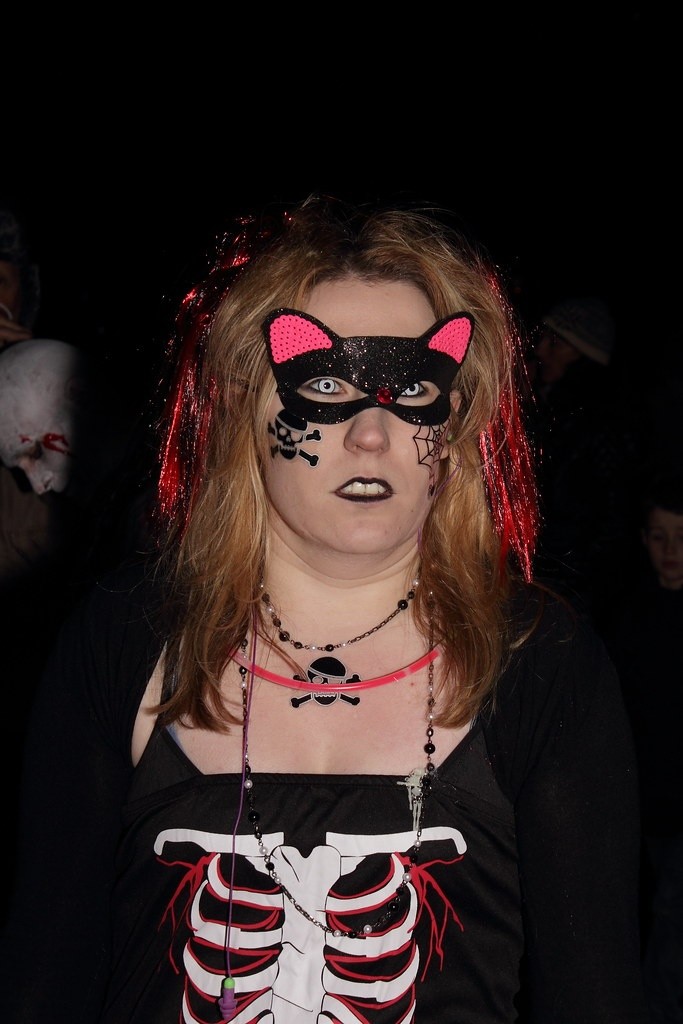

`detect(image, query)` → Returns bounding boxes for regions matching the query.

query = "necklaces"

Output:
[224,627,456,694]
[241,627,436,941]
[257,578,421,651]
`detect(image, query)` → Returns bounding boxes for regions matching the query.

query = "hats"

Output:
[541,298,614,367]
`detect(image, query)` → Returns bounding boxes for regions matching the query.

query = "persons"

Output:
[0,204,682,1024]
